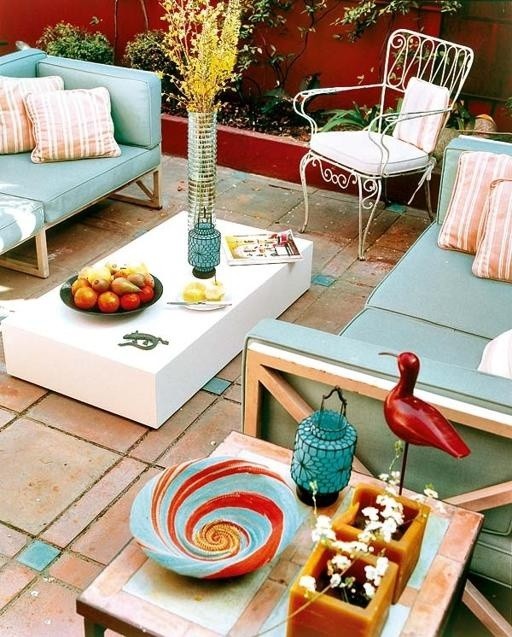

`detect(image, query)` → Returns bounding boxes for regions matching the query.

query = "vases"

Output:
[287,540,399,635]
[326,479,431,606]
[184,110,219,234]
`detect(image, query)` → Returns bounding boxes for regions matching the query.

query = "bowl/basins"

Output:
[130,456,303,579]
[60,267,165,316]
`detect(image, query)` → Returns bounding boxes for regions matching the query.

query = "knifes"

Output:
[167,301,232,307]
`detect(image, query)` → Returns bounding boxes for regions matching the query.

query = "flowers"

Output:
[279,478,393,607]
[333,440,438,553]
[152,0,257,214]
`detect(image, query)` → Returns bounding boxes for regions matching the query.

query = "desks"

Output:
[2,209,313,429]
[79,439,485,636]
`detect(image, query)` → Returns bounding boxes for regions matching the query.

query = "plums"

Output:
[71,279,155,312]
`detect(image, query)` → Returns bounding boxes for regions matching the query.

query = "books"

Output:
[224,229,304,267]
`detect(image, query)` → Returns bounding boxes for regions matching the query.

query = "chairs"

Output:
[299,25,475,262]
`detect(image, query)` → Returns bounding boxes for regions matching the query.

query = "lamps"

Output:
[186,202,224,279]
[290,385,357,506]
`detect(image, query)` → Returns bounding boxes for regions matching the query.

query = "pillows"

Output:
[0,73,65,154]
[471,177,512,284]
[19,85,122,164]
[437,149,512,255]
[392,72,452,154]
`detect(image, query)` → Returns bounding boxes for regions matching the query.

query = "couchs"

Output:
[238,132,511,636]
[0,46,165,279]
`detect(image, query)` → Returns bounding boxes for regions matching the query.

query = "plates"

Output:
[185,302,225,311]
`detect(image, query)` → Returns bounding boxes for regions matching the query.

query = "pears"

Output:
[87,261,154,294]
